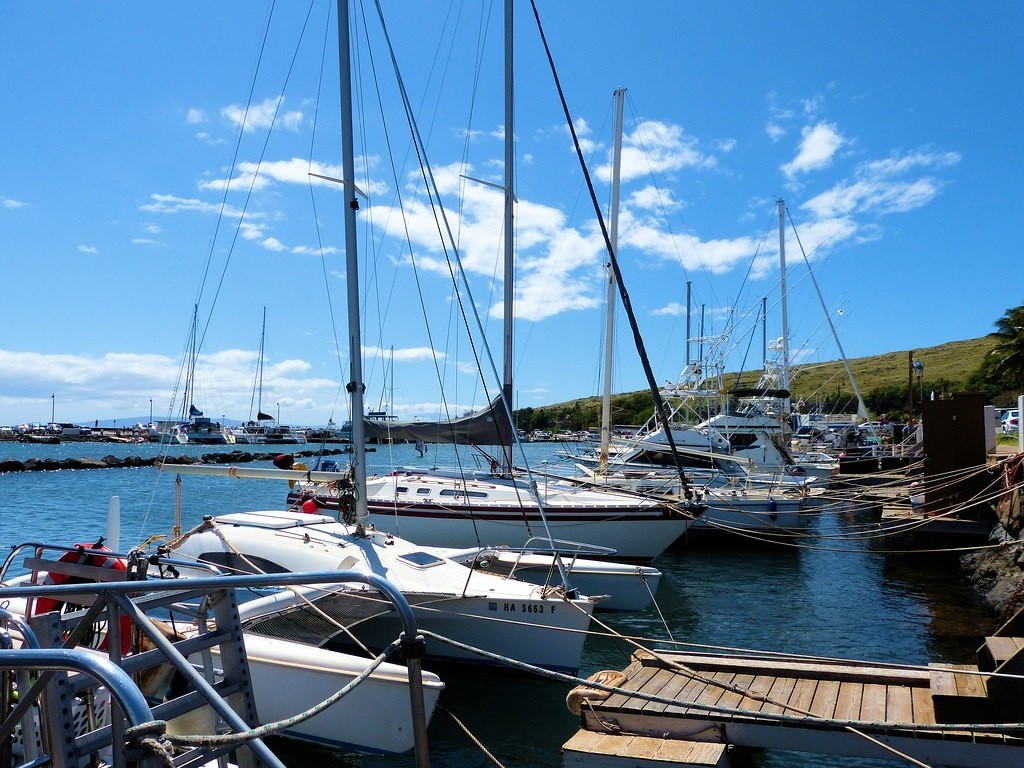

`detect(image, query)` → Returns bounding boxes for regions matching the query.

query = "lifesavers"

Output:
[34,543,132,660]
[38,431,42,435]
[342,495,356,523]
[303,430,309,438]
[45,431,49,435]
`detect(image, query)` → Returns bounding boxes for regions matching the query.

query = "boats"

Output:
[126,436,145,444]
[0,557,444,758]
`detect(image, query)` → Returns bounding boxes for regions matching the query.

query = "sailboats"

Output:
[543,82,882,527]
[0,0,598,670]
[288,0,703,560]
[157,303,307,446]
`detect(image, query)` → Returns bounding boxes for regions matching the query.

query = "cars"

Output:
[859,421,881,430]
[1001,410,1019,434]
